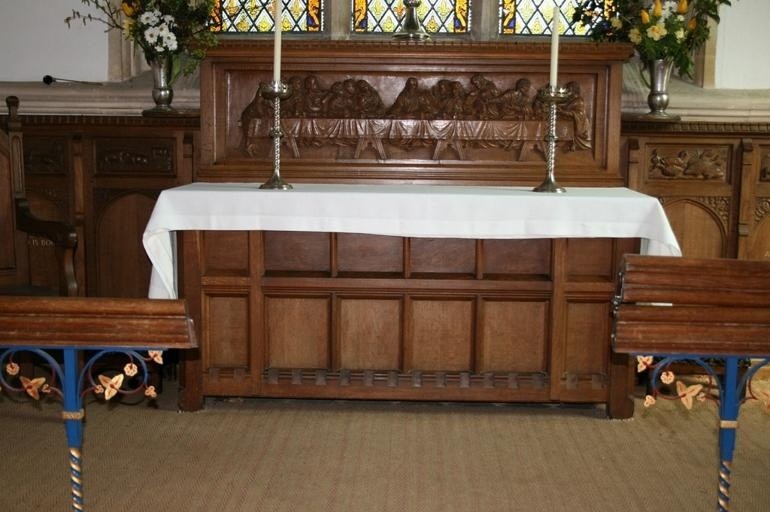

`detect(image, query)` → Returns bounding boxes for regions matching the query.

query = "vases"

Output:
[143,54,178,114]
[645,59,675,120]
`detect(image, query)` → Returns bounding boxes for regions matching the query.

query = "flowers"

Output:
[572,0,731,89]
[63,0,218,87]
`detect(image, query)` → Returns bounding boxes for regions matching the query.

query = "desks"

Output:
[156,183,657,419]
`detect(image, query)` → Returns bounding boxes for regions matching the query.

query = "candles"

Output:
[273,0,282,81]
[549,5,561,87]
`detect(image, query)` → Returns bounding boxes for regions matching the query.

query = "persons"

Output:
[241,72,593,162]
